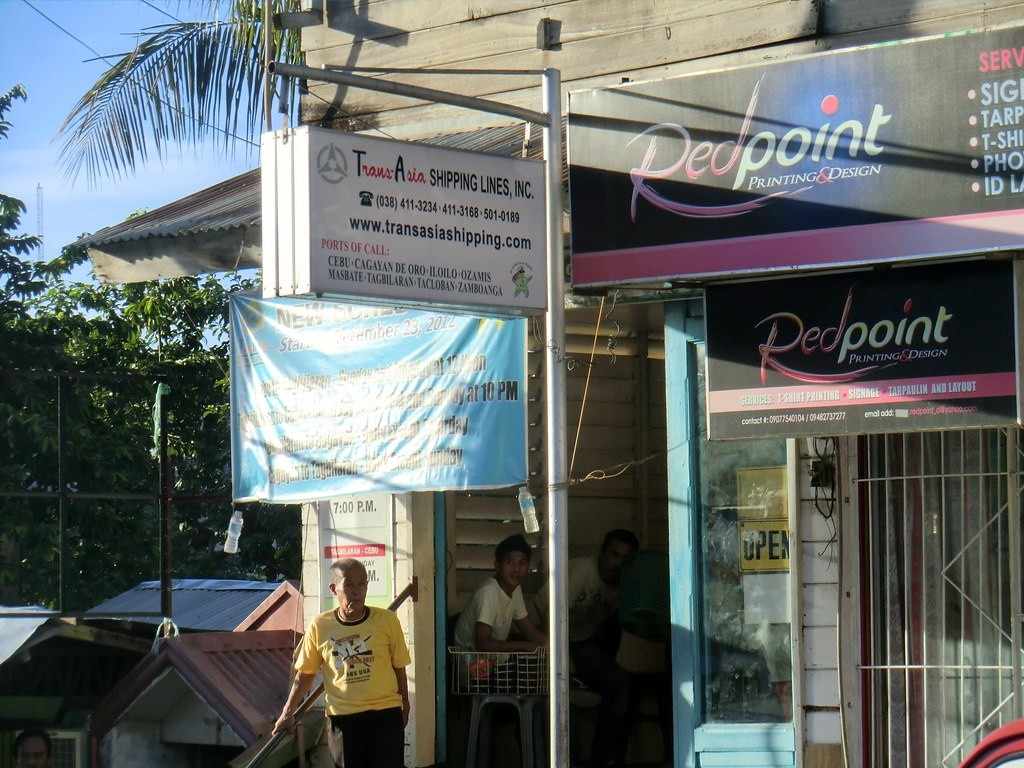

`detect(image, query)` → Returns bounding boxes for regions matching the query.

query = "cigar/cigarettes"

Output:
[348,601,353,609]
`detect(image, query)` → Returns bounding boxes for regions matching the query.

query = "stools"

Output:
[466,693,549,768]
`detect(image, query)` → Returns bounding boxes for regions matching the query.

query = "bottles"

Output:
[223,510,244,553]
[518,486,540,533]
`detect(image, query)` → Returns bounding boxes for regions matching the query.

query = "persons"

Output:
[272,559,411,768]
[529,529,671,768]
[453,534,600,707]
[13,728,53,768]
